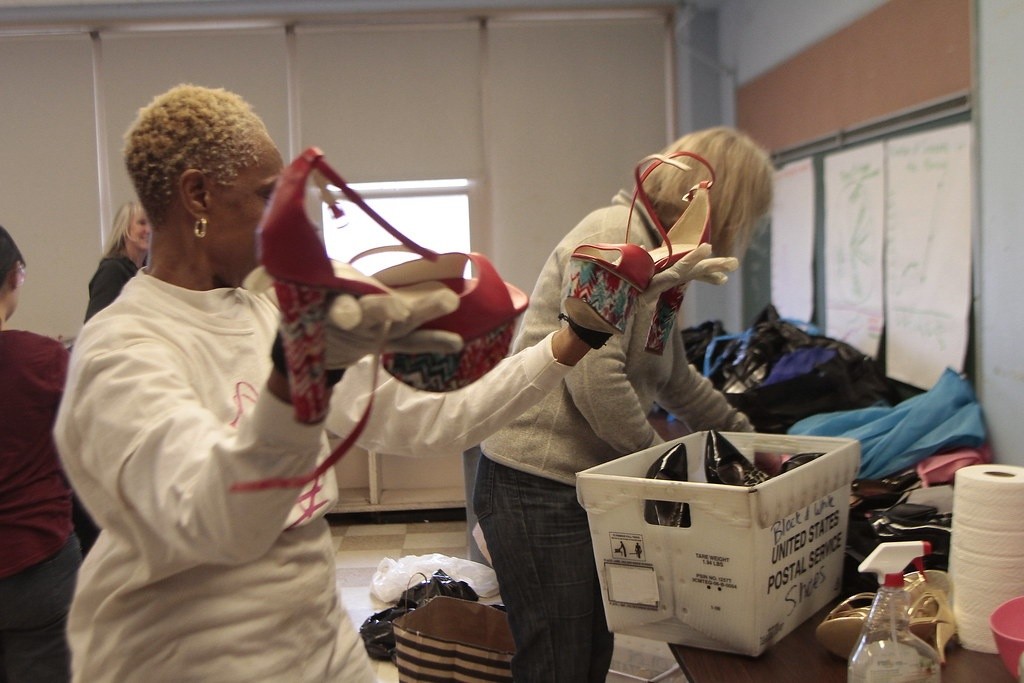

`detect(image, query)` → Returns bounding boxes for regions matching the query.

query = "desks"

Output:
[665,600,1024,683]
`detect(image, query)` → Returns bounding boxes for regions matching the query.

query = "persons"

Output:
[470,124,775,683]
[0,225,102,683]
[82,202,155,322]
[50,92,741,683]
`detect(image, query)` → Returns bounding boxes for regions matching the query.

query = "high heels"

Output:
[228,145,529,492]
[815,578,955,666]
[561,152,716,356]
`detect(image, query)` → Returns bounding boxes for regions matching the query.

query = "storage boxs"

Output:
[571,430,863,662]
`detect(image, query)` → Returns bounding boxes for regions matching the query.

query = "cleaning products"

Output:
[846,540,944,682]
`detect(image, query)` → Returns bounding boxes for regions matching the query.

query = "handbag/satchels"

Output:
[391,571,516,683]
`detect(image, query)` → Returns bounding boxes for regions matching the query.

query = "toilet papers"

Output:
[950,463,1024,654]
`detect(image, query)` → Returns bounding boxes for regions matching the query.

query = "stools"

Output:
[390,594,522,683]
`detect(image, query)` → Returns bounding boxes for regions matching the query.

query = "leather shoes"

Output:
[642,430,828,527]
[846,470,953,577]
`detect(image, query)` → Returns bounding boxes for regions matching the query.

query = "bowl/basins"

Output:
[987,595,1024,682]
